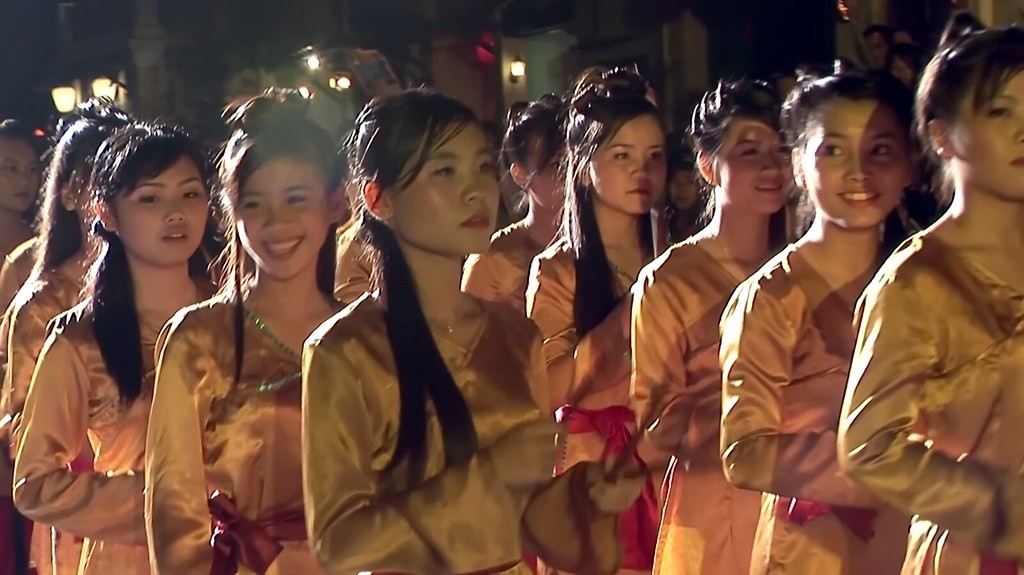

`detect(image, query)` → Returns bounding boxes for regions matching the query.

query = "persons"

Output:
[0,61,669,575]
[627,11,1024,575]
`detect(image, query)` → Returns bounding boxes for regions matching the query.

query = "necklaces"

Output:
[429,300,460,333]
[610,260,639,287]
